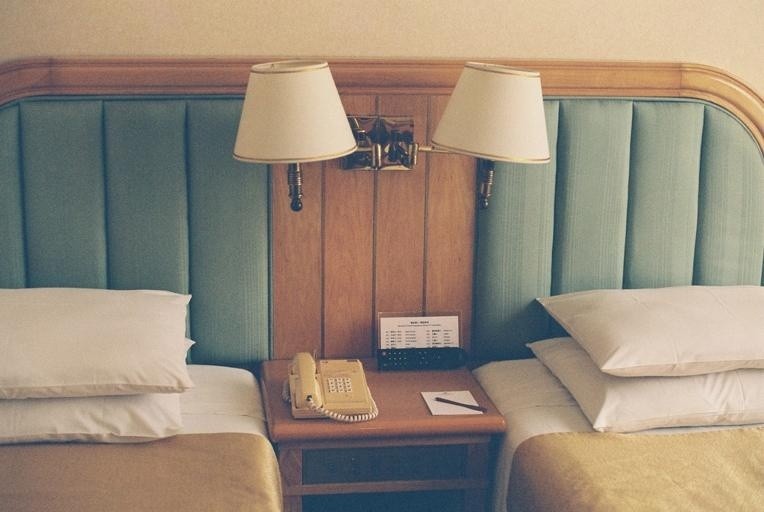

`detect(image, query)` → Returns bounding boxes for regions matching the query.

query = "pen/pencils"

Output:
[435,397,489,412]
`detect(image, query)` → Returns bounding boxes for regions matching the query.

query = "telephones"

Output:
[282,352,378,422]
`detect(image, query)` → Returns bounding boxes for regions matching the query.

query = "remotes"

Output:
[376,347,469,371]
